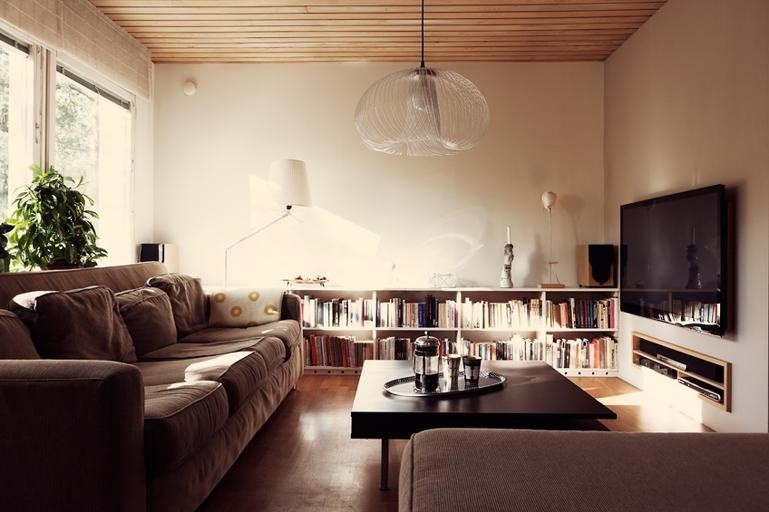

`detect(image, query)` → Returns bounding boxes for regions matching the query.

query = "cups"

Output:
[441,354,482,384]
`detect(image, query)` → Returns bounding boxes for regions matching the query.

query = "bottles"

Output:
[414,330,440,391]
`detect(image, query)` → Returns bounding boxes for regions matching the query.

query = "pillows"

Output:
[2,273,285,378]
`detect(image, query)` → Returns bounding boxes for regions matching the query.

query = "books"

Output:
[304,333,374,368]
[301,295,373,328]
[461,296,542,328]
[376,336,457,360]
[545,333,620,371]
[546,293,617,328]
[460,336,543,361]
[377,294,457,327]
[638,298,720,332]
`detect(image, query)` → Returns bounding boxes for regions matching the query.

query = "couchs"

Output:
[1,258,309,511]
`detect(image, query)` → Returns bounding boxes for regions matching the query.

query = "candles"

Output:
[507,225,512,244]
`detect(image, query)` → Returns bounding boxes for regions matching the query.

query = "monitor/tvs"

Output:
[620,183,729,340]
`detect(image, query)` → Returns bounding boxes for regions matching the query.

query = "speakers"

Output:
[576,243,616,287]
[138,243,178,273]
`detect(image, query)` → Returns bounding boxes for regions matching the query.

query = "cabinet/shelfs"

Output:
[288,287,618,377]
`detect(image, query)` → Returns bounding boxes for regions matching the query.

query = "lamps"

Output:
[221,159,312,297]
[349,1,490,161]
